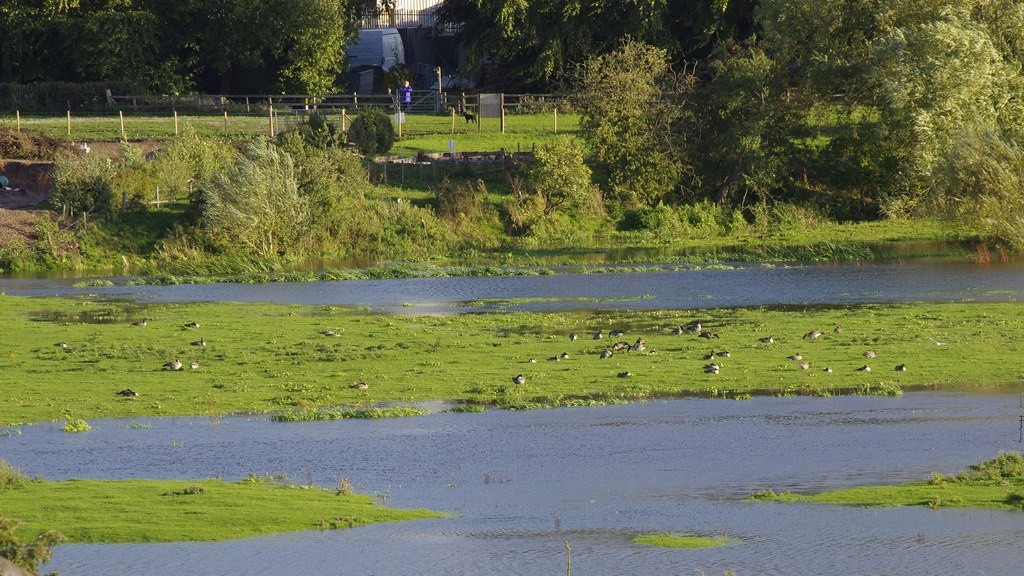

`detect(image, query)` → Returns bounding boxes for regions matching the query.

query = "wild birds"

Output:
[53,342,66,347]
[190,337,205,346]
[115,389,138,397]
[351,382,367,390]
[163,359,182,371]
[131,318,146,326]
[324,330,340,337]
[185,321,199,328]
[511,322,906,384]
[190,360,199,369]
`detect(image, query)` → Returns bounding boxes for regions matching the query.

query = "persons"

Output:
[385,88,394,114]
[400,81,412,114]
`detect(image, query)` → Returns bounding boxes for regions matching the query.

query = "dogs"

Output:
[463,112,477,124]
